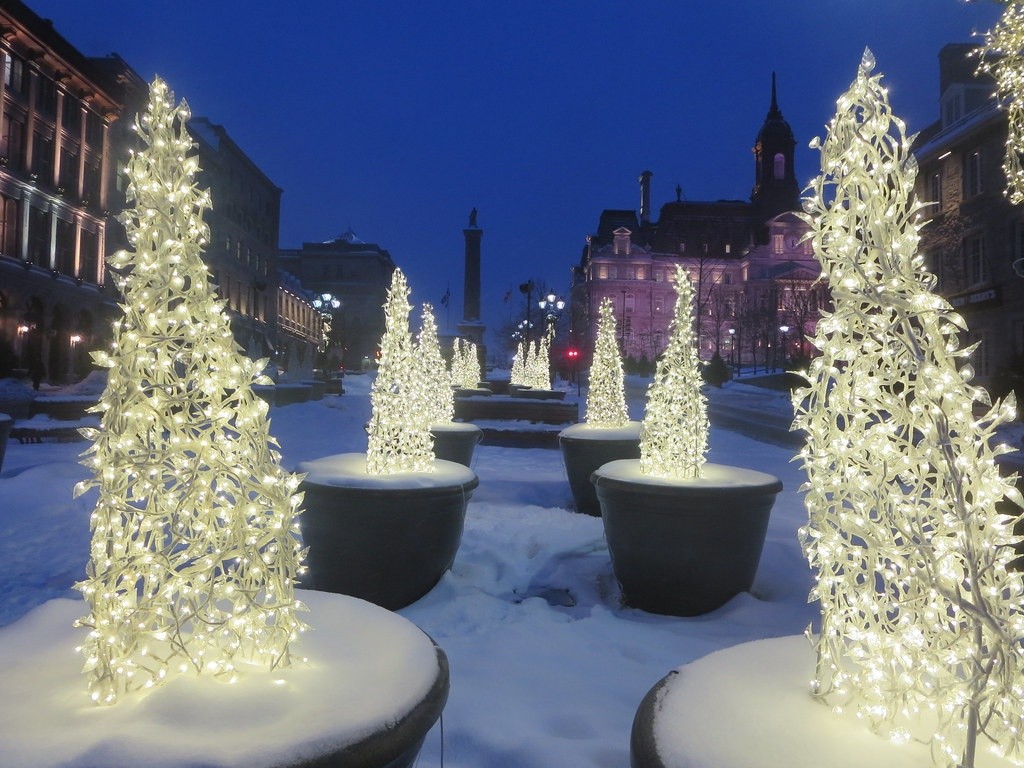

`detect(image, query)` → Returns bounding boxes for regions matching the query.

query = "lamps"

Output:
[70,336,80,347]
[17,326,28,337]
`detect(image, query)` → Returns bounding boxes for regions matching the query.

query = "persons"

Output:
[469,206,478,229]
[560,362,575,382]
[31,360,46,391]
[675,183,683,201]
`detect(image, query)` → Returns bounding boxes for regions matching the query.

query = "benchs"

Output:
[13,425,85,444]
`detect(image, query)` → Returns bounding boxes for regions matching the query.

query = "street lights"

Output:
[780,320,790,372]
[729,323,736,366]
[538,287,566,374]
[312,291,340,378]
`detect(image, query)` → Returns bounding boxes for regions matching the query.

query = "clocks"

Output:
[785,235,800,251]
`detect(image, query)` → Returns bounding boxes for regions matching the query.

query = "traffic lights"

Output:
[567,350,578,358]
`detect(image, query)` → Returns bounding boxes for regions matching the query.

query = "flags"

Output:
[503,289,512,302]
[440,288,451,307]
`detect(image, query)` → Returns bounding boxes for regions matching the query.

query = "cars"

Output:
[312,360,344,379]
